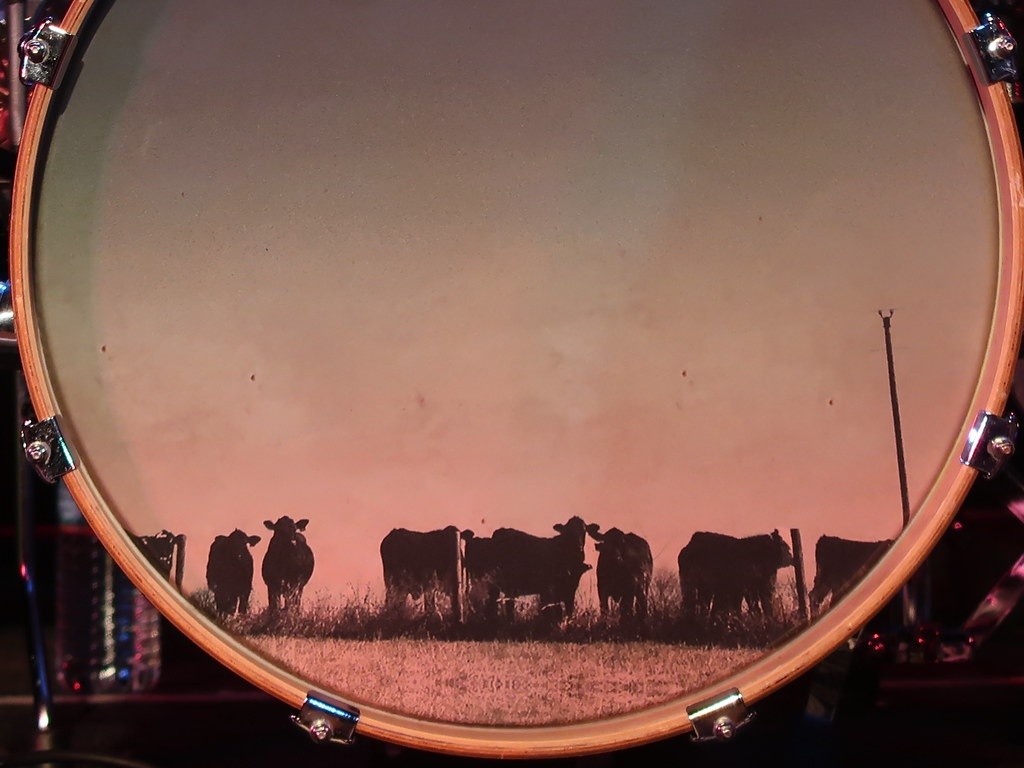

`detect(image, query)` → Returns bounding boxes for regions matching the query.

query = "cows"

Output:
[122,515,895,638]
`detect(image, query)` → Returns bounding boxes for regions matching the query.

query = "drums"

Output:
[6,0,1024,760]
